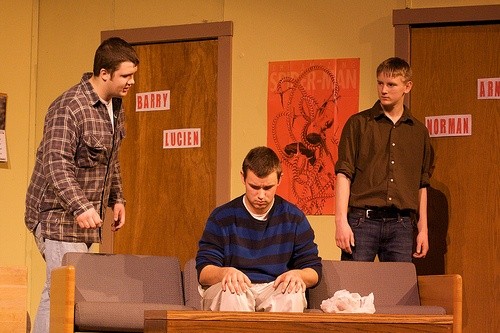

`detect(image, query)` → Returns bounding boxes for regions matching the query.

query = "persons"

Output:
[333,57,437,263]
[24,37,139,331]
[193,146,323,312]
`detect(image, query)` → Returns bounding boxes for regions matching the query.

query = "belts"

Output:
[349,208,412,218]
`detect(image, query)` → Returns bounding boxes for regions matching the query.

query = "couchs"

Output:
[50,252,462,333]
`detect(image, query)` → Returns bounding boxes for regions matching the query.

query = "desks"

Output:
[144,310,454,333]
